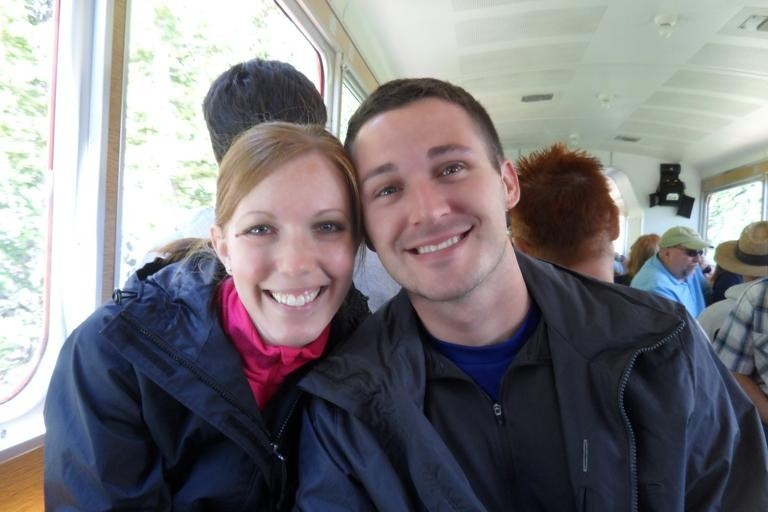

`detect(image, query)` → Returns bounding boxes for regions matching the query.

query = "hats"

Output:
[659,226,713,250]
[714,221,768,278]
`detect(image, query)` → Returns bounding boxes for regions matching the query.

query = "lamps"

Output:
[567,133,582,152]
[651,14,679,38]
[595,89,619,113]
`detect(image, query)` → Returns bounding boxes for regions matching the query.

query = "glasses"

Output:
[676,246,703,257]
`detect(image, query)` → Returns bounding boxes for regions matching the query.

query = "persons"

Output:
[614,223,715,321]
[42,113,378,511]
[697,220,768,346]
[711,274,768,446]
[271,77,768,511]
[201,52,404,315]
[505,138,621,287]
